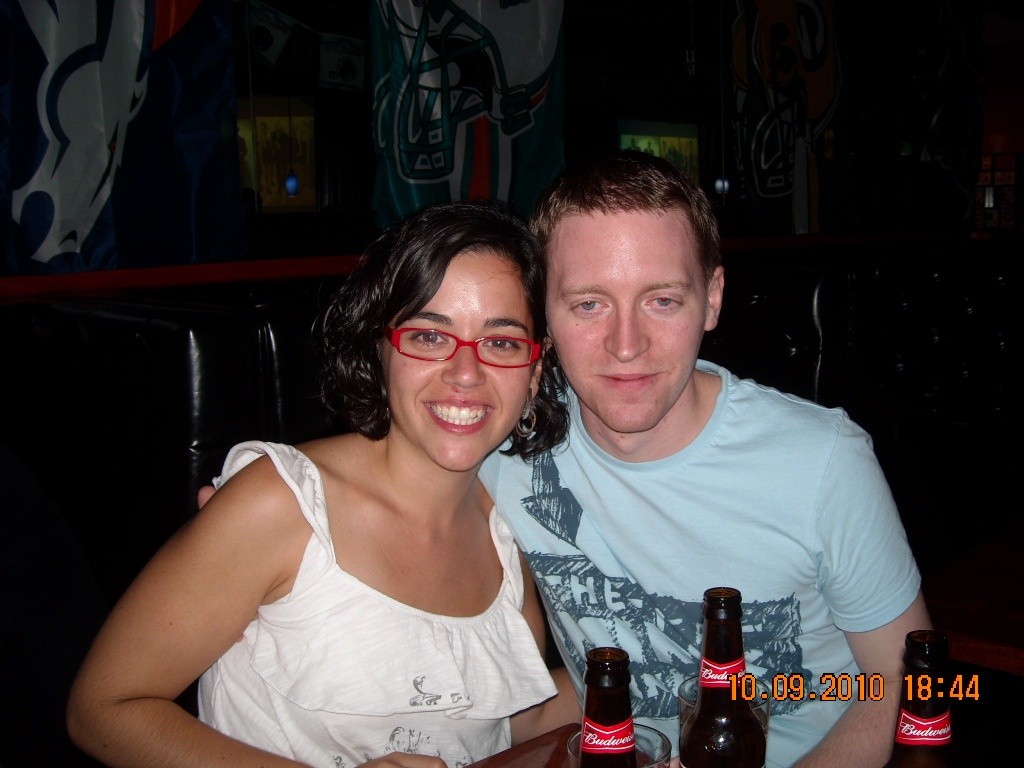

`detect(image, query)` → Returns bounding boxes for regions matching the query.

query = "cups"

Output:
[678,676,771,740]
[567,724,670,768]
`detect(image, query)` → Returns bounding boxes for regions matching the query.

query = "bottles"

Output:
[892,630,961,768]
[578,646,639,768]
[680,587,767,768]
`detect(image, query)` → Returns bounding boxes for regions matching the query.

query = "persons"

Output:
[67,203,584,768]
[198,149,932,767]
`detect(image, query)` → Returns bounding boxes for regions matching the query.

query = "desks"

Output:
[923,519,1024,676]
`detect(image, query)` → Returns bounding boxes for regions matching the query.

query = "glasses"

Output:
[382,325,542,367]
